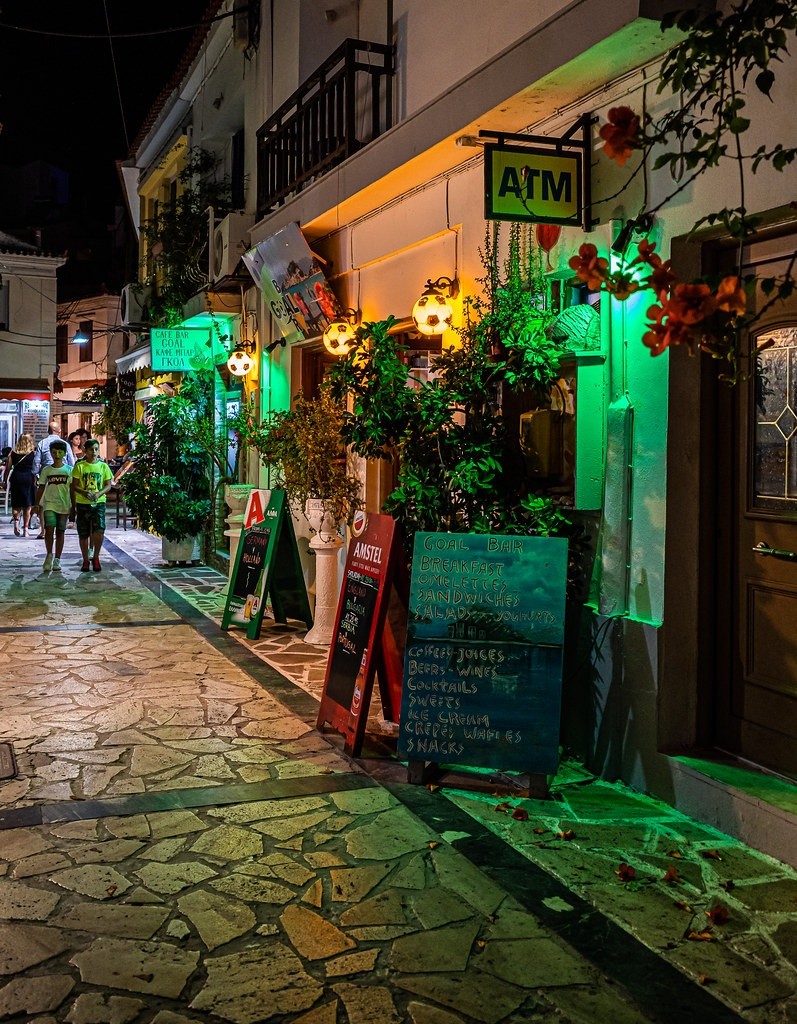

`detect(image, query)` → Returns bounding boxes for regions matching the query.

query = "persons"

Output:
[31,440,76,571]
[69,439,114,572]
[0,422,105,560]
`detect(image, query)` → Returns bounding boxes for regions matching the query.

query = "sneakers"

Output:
[43,553,53,570]
[52,558,61,570]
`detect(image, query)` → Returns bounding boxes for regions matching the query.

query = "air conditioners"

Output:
[121,284,152,327]
[213,213,255,288]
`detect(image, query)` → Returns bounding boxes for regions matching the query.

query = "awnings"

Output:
[0,377,51,401]
[114,338,152,377]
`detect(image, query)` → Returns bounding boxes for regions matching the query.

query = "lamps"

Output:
[322,308,363,356]
[226,340,256,376]
[70,330,89,344]
[262,337,286,357]
[412,277,460,336]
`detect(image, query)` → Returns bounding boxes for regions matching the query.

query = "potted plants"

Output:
[122,369,213,566]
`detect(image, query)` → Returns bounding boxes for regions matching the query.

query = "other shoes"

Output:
[24,528,30,537]
[80,560,90,571]
[14,520,20,536]
[37,533,44,538]
[67,522,74,528]
[90,558,101,572]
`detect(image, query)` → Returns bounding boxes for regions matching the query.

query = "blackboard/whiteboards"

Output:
[326,510,394,721]
[399,536,572,774]
[223,489,287,626]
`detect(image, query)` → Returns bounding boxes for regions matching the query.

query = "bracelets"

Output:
[34,504,40,508]
